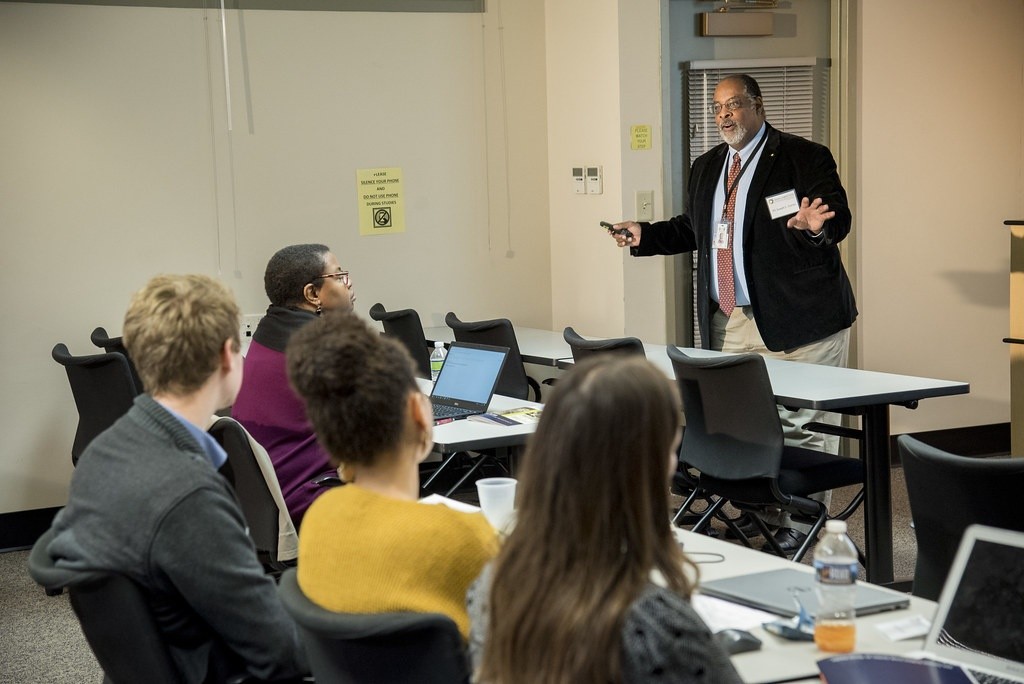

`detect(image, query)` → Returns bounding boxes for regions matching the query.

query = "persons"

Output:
[287,312,502,642]
[232,243,356,534]
[469,354,743,684]
[26,276,311,684]
[718,233,724,243]
[607,74,859,556]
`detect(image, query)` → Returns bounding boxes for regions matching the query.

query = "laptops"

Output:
[917,524,1024,684]
[699,568,911,625]
[429,341,510,421]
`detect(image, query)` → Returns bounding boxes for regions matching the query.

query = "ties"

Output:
[717,152,742,318]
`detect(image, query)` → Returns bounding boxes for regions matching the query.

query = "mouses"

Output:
[712,630,761,657]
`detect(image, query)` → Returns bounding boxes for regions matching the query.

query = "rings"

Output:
[817,209,822,213]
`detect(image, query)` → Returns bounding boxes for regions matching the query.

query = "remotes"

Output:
[600,221,633,238]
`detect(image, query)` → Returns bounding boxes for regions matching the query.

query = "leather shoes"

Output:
[762,527,819,561]
[724,511,780,540]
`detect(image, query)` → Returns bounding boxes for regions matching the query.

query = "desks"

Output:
[648,526,938,683]
[380,327,604,365]
[413,379,546,477]
[557,346,970,583]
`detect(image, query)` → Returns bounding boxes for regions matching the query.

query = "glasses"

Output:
[303,267,348,292]
[714,95,756,114]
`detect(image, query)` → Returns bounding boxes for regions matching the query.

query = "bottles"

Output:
[430,342,447,386]
[815,520,859,651]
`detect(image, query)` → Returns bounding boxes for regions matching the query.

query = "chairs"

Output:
[444,312,540,403]
[562,325,865,561]
[369,302,432,378]
[68,573,179,684]
[897,433,1024,602]
[282,568,470,684]
[51,327,145,467]
[209,418,299,574]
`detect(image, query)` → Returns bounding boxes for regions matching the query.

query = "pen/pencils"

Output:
[434,418,455,426]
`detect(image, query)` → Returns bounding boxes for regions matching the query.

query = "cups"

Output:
[475,478,518,529]
